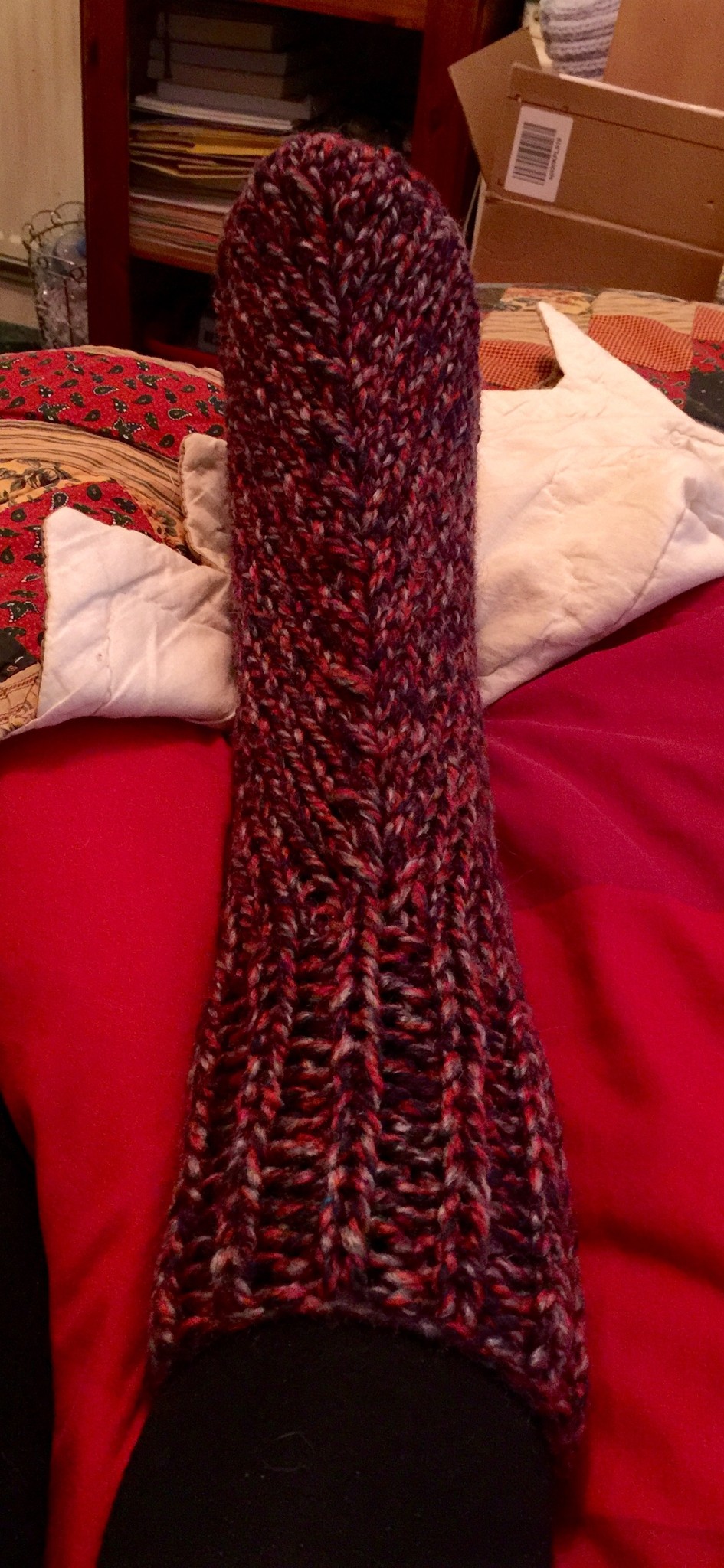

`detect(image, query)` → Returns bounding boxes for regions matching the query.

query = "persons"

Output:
[0,128,586,1568]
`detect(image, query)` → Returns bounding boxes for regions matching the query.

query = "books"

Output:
[130,54,310,274]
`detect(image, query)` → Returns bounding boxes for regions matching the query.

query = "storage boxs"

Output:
[465,61,724,306]
